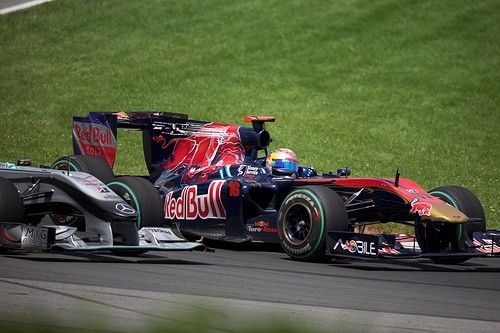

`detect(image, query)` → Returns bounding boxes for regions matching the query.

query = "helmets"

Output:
[266,148,299,176]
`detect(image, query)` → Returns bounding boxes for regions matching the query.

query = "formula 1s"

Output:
[71,111,500,265]
[1,155,211,258]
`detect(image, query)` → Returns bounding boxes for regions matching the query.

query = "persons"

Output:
[266,148,315,178]
